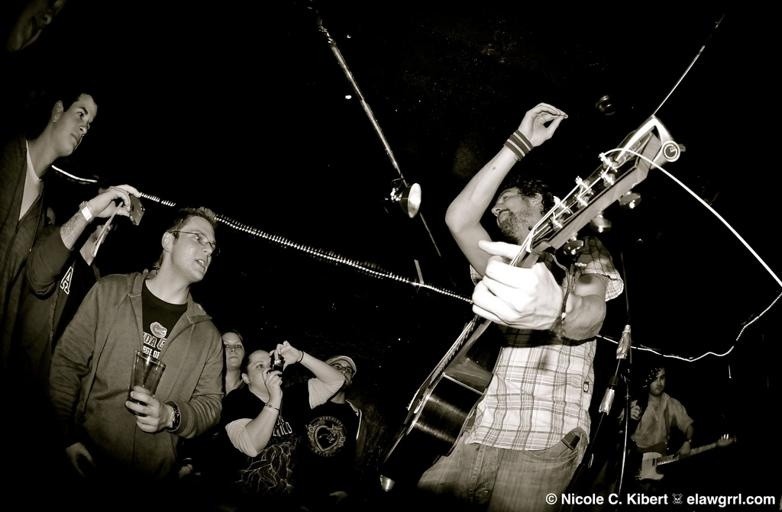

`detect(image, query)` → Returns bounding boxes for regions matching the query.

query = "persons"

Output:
[415,102,629,511]
[617,365,698,475]
[0,0,390,509]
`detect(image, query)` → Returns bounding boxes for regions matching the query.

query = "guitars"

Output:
[382,115,686,492]
[635,433,736,481]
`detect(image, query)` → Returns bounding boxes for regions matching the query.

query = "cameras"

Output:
[130,195,146,225]
[271,353,284,373]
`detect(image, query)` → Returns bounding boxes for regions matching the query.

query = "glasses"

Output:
[171,231,220,254]
[332,363,353,374]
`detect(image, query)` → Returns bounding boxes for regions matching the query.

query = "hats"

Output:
[326,355,357,378]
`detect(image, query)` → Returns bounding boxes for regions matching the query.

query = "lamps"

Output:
[382,174,425,224]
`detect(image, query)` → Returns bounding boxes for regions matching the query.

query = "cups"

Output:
[124,351,165,415]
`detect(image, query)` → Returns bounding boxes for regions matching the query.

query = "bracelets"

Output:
[503,130,535,163]
[684,438,693,443]
